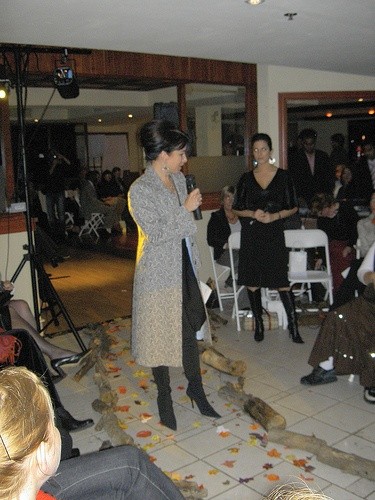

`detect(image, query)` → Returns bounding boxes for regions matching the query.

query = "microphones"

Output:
[184,174,203,220]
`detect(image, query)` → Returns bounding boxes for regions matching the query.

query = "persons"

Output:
[289,129,375,311]
[0,282,187,500]
[300,241,375,404]
[232,133,304,343]
[264,477,335,500]
[207,185,254,317]
[38,145,128,242]
[128,120,221,429]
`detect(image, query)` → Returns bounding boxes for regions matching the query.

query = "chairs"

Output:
[190,209,336,332]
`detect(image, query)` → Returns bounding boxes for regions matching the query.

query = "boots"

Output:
[248,286,264,342]
[182,337,222,418]
[279,287,305,344]
[152,365,177,431]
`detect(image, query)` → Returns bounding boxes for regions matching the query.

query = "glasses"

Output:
[223,196,235,200]
[254,147,267,152]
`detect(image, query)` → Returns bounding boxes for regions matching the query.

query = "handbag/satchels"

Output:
[243,304,278,330]
[304,265,328,302]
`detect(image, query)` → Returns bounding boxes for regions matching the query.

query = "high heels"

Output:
[50,253,71,267]
[50,348,92,383]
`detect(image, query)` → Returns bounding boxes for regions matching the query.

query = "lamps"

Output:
[52,57,80,99]
[0,82,12,98]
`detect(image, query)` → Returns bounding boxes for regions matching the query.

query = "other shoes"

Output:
[364,385,375,404]
[67,447,80,459]
[225,274,233,287]
[299,365,337,384]
[59,410,95,435]
